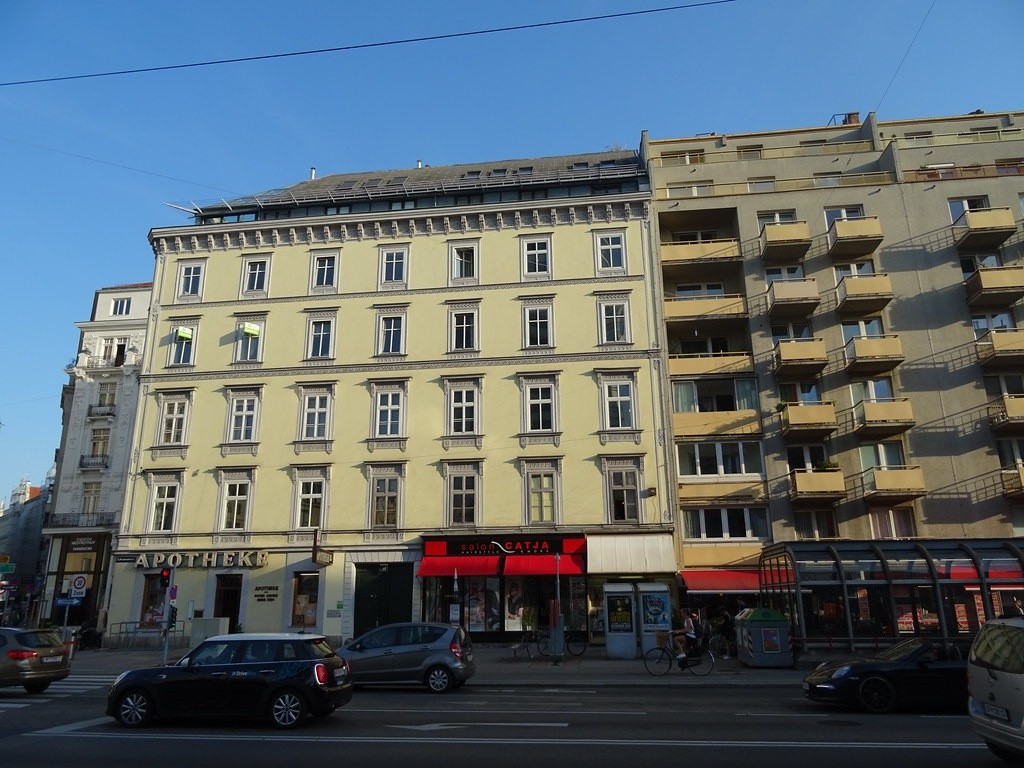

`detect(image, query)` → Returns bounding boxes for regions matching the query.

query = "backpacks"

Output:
[689,618,703,638]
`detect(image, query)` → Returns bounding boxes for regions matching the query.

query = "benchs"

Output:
[506,632,531,660]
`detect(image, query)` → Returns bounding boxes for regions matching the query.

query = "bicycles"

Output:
[644,630,715,677]
[537,623,587,657]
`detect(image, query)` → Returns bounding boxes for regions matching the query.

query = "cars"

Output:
[801,638,973,713]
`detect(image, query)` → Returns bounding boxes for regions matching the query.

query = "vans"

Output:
[967,618,1024,762]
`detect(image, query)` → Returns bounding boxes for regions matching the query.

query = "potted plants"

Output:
[918,164,938,180]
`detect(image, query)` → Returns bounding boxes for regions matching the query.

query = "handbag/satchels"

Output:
[720,624,731,632]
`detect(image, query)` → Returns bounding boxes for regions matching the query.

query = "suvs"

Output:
[0,627,70,694]
[334,622,475,694]
[104,633,356,729]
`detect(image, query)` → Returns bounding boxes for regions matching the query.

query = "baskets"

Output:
[712,626,720,634]
[656,632,668,648]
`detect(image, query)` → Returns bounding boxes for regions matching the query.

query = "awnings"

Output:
[675,569,812,595]
[584,532,679,574]
[416,554,584,589]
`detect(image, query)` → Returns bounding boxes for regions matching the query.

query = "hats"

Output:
[718,605,727,611]
[689,614,698,620]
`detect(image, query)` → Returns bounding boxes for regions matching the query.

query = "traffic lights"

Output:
[158,567,171,588]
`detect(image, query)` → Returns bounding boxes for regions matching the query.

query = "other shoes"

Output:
[718,654,731,659]
[681,664,687,671]
[676,653,686,659]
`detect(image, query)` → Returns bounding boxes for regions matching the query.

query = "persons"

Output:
[713,607,735,659]
[506,584,523,619]
[668,608,696,660]
